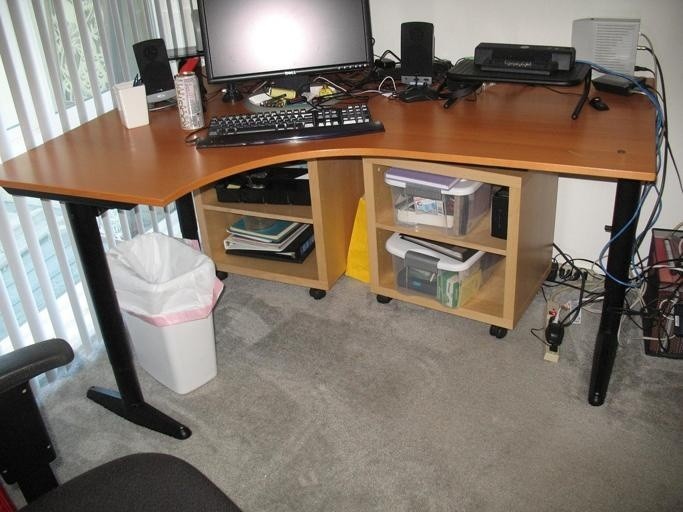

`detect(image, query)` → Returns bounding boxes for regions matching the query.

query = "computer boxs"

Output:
[642,228,683,359]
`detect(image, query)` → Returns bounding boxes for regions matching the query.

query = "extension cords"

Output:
[549,267,595,281]
[364,63,401,81]
[545,301,561,361]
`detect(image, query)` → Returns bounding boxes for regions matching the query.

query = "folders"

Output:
[226,224,314,264]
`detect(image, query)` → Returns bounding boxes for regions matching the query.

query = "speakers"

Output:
[132,38,178,111]
[400,21,435,86]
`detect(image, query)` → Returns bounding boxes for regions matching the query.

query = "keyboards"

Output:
[197,102,385,150]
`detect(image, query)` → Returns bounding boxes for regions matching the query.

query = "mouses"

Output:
[399,86,437,101]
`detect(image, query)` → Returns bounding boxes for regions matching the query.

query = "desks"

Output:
[0,60,663,439]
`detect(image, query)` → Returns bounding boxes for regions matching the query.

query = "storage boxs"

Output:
[384,232,498,309]
[384,168,494,237]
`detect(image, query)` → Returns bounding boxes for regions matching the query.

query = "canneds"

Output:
[174,72,205,131]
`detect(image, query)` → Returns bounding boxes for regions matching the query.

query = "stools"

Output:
[0,335,246,512]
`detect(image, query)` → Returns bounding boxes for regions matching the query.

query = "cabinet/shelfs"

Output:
[363,157,558,338]
[191,157,366,300]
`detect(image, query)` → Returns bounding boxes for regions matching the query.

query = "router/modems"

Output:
[572,17,641,76]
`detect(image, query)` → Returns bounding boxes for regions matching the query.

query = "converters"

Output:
[376,59,396,70]
[547,262,559,282]
[545,323,565,346]
[673,303,683,337]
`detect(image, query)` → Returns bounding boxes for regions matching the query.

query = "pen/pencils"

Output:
[133,73,144,86]
[261,93,287,105]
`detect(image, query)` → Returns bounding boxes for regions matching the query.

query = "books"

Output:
[384,168,493,308]
[223,216,315,264]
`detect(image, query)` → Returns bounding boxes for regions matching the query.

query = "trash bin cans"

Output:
[105,231,223,394]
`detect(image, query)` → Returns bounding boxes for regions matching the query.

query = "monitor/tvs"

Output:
[197,0,374,111]
